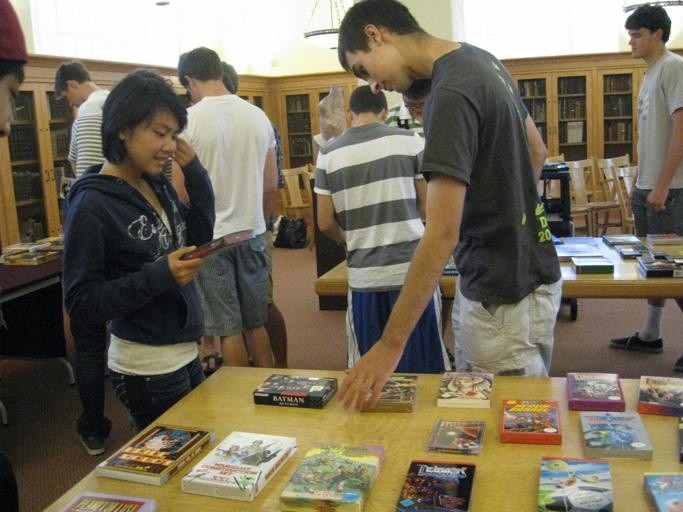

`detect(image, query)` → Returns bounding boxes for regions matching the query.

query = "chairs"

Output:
[279,164,317,250]
[541,153,639,240]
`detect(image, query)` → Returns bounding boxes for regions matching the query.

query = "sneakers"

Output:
[609,331,662,353]
[80,414,112,456]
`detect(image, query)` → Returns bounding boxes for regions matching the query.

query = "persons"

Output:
[383,80,431,143]
[313,85,453,373]
[0,0,30,512]
[56,47,286,454]
[610,4,683,371]
[337,1,564,413]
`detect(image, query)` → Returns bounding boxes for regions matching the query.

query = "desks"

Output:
[43,366,683,511]
[315,232,683,298]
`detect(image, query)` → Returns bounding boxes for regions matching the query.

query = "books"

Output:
[443,257,459,276]
[0,89,75,266]
[638,376,682,416]
[287,96,313,165]
[254,374,336,409]
[280,443,384,512]
[428,419,486,455]
[537,456,613,512]
[502,399,561,445]
[361,375,417,412]
[644,472,683,512]
[518,78,635,142]
[579,410,653,460]
[58,492,157,512]
[551,233,683,278]
[397,460,476,512]
[437,372,492,408]
[566,372,625,412]
[182,430,297,502]
[96,422,216,487]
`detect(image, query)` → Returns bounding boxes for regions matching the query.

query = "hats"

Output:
[0,1,28,62]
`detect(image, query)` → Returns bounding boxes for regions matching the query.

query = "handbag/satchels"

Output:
[273,216,307,248]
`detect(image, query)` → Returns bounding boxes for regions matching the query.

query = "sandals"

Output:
[201,351,222,377]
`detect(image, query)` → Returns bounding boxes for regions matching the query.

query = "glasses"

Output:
[402,101,422,110]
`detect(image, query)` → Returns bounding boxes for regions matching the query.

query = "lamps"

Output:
[300,1,357,50]
[619,0,683,53]
